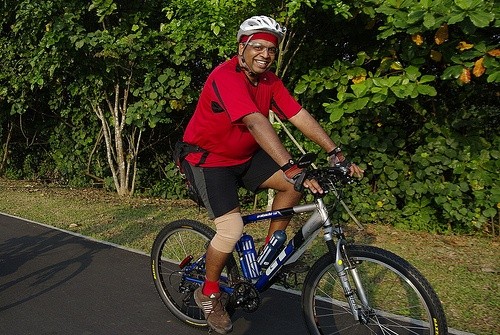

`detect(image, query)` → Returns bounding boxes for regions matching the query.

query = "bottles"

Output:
[257,230,287,269]
[236,233,260,279]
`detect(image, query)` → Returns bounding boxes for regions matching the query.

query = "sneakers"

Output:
[194,282,234,334]
[257,245,311,273]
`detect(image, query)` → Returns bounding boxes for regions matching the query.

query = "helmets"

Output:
[237,16,287,43]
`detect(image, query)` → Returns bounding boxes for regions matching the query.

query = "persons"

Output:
[181,15,363,335]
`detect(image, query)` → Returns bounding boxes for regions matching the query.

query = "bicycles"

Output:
[151,142,449,335]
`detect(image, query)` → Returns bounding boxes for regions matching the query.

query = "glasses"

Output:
[240,42,278,54]
